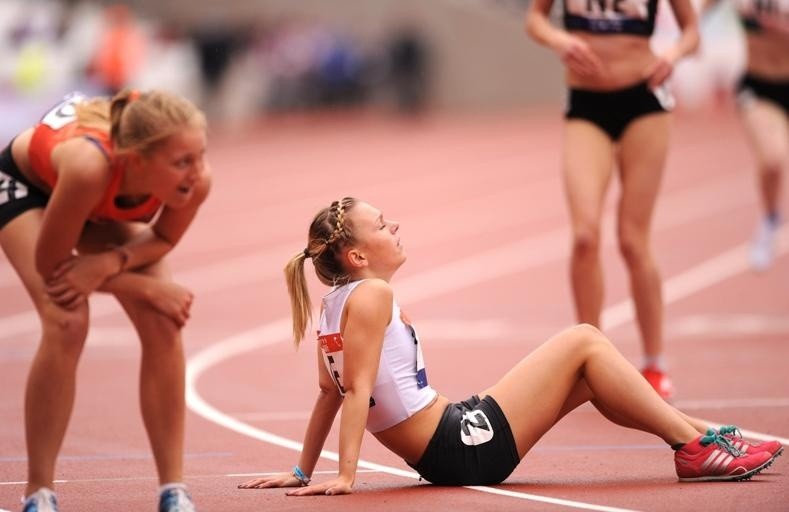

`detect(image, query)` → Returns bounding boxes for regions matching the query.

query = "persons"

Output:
[522,0,706,405]
[0,82,218,512]
[236,195,786,496]
[697,0,788,271]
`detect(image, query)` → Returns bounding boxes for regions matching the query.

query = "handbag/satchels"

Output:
[294,466,311,486]
[106,246,128,274]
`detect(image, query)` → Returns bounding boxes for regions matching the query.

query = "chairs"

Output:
[155,481,197,512]
[748,216,781,273]
[671,425,784,482]
[18,487,58,512]
[639,359,674,399]
